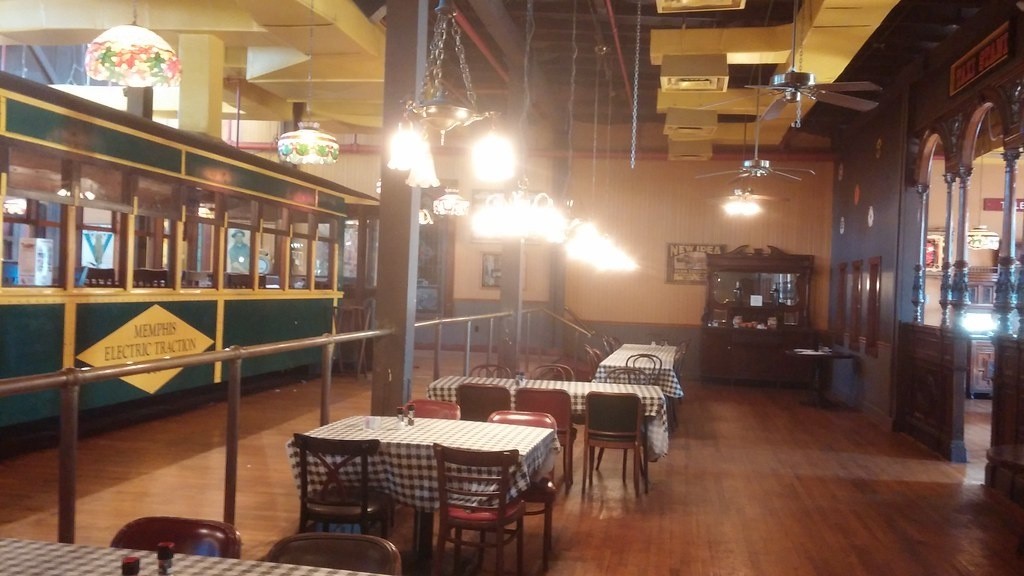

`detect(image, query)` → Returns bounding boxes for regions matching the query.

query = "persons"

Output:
[229,230,249,272]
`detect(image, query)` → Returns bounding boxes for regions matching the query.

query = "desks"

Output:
[428,374,666,494]
[784,347,857,408]
[592,343,682,432]
[0,536,387,576]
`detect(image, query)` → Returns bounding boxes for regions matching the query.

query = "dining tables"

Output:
[284,415,561,576]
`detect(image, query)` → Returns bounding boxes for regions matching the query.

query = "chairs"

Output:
[516,386,577,493]
[82,266,375,381]
[293,432,393,540]
[264,533,401,576]
[673,338,691,403]
[486,410,557,572]
[429,442,524,576]
[626,353,662,385]
[469,363,511,378]
[530,363,574,380]
[604,368,650,384]
[582,391,642,496]
[110,516,242,560]
[401,398,461,419]
[584,334,622,373]
[457,383,511,421]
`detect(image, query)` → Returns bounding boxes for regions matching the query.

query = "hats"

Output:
[231,230,245,238]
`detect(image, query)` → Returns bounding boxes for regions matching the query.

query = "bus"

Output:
[0,68,381,428]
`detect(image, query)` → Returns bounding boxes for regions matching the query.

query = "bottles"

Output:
[407,405,415,429]
[397,407,405,431]
[520,372,525,387]
[733,280,743,305]
[157,541,176,576]
[515,371,520,385]
[122,557,140,576]
[769,283,779,307]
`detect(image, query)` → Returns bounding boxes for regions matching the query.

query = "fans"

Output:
[695,63,817,184]
[744,0,882,121]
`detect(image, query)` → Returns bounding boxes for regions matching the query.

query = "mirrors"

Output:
[706,261,809,312]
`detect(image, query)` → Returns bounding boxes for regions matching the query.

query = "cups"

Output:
[363,415,381,433]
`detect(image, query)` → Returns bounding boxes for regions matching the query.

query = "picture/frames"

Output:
[478,251,501,289]
[925,228,946,279]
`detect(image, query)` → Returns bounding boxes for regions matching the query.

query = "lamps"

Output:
[387,0,516,188]
[966,155,999,250]
[84,0,181,87]
[276,0,340,164]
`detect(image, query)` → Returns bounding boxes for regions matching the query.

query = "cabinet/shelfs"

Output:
[968,340,995,399]
[700,327,816,385]
[967,267,1023,310]
